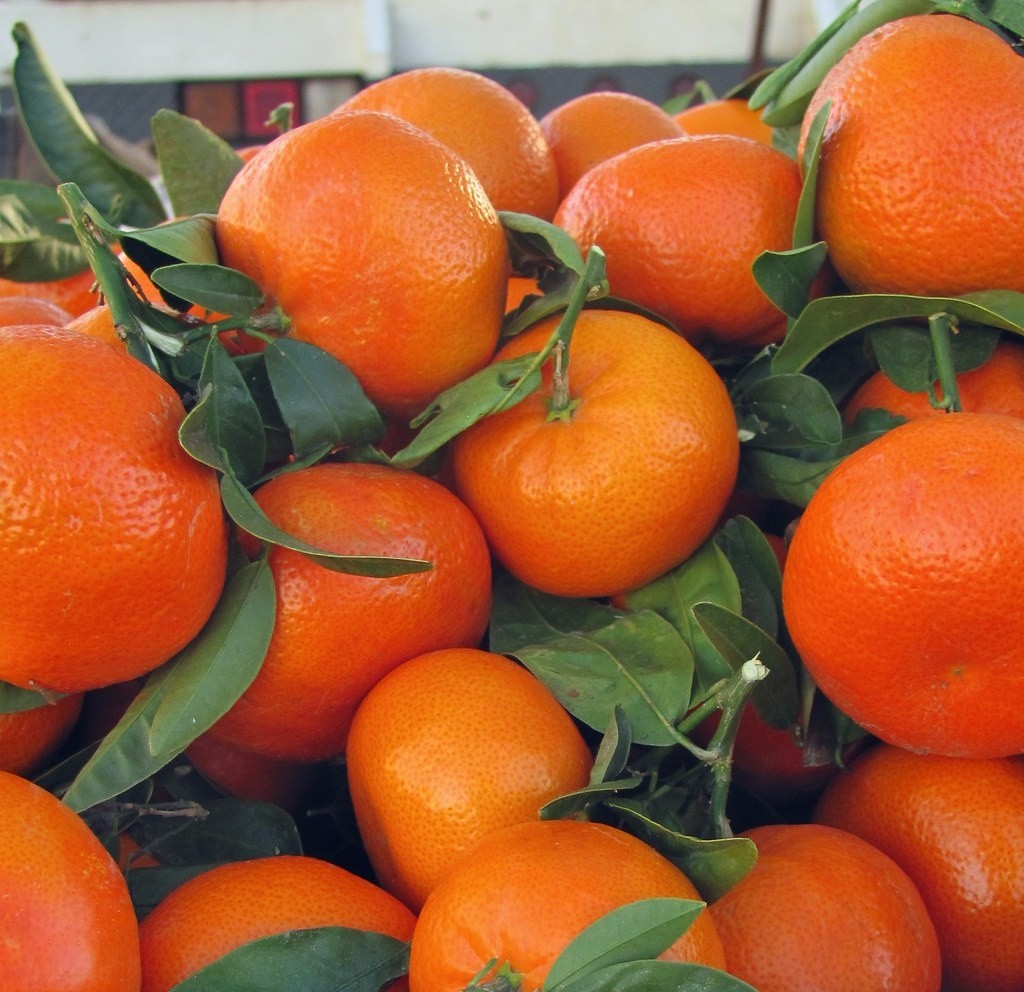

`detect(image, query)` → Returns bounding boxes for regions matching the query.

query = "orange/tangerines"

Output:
[0,14,1024,992]
